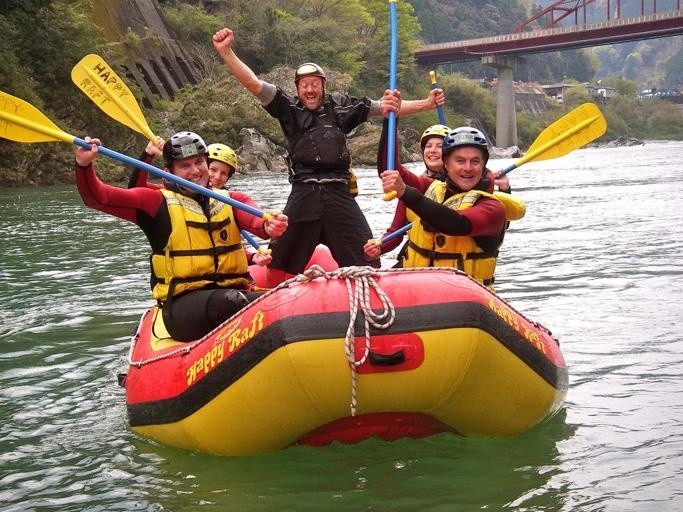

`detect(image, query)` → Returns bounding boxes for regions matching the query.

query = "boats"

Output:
[125,245,570,458]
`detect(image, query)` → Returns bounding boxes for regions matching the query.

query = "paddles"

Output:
[71,54,272,257]
[1,91,282,225]
[368,102,607,246]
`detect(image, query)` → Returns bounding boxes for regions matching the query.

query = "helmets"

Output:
[418,124,489,166]
[163,131,238,173]
[295,63,326,86]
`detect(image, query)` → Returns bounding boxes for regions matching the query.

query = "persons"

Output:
[127,134,272,267]
[376,88,507,296]
[210,25,445,276]
[74,130,288,342]
[362,121,512,268]
